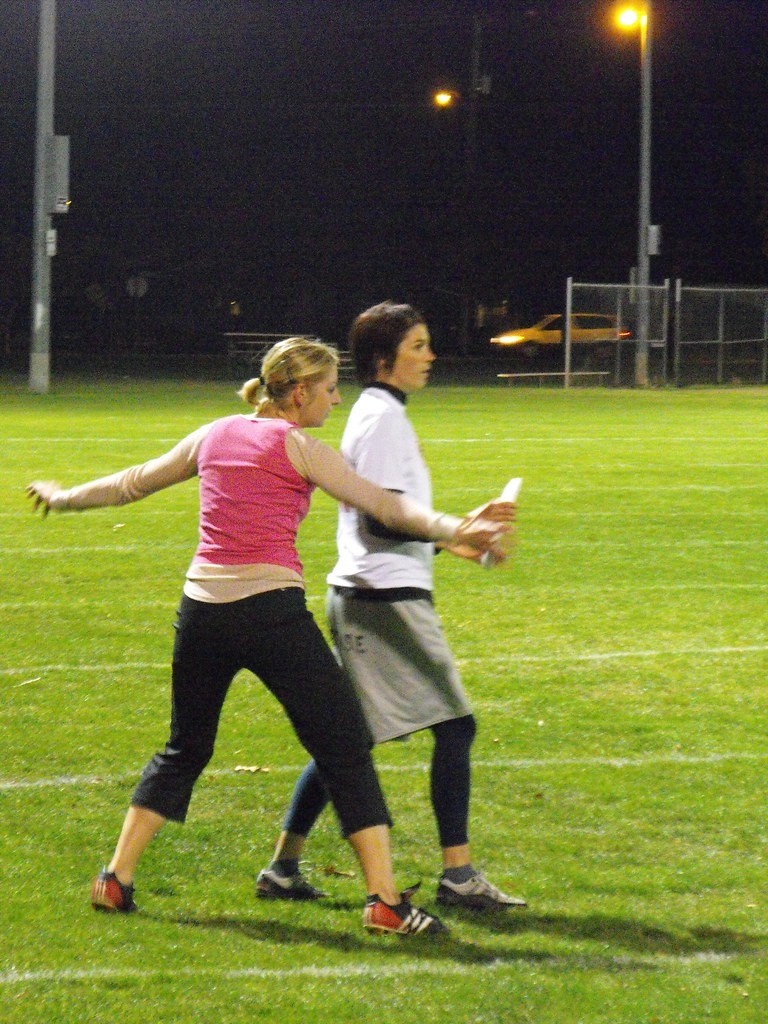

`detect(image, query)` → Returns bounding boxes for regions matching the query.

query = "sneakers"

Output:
[362,882,450,938]
[254,870,326,901]
[436,874,527,912]
[91,865,137,914]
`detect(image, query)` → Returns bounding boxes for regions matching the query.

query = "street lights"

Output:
[614,0,650,388]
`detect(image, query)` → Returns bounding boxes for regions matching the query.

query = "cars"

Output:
[489,311,634,372]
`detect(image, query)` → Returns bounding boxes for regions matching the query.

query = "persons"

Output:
[25,338,516,935]
[254,301,529,912]
